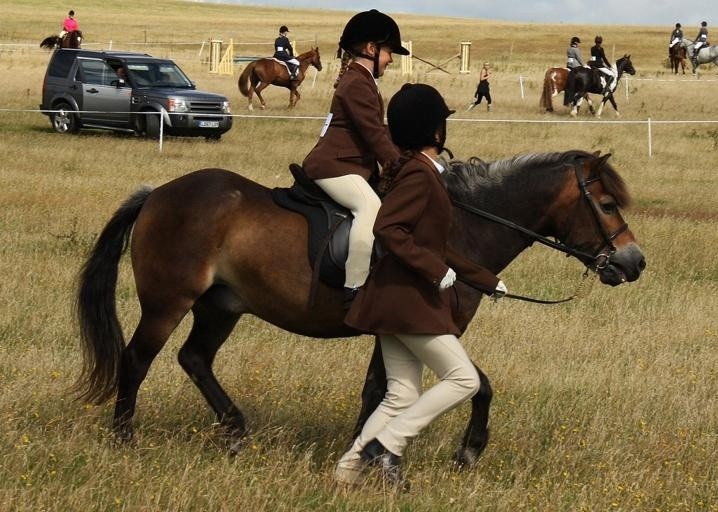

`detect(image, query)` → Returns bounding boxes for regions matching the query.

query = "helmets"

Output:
[676,23,681,27]
[387,83,456,147]
[595,36,603,44]
[701,21,707,26]
[279,26,289,33]
[571,37,582,44]
[69,10,74,15]
[342,9,410,55]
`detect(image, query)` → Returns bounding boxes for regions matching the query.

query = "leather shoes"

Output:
[343,287,359,310]
[360,437,410,491]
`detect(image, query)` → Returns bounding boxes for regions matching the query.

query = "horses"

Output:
[560,53,637,119]
[38,30,84,49]
[667,40,686,75]
[70,147,648,470]
[676,38,718,75]
[539,66,596,115]
[237,46,322,110]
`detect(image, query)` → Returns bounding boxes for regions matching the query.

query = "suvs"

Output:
[40,49,233,141]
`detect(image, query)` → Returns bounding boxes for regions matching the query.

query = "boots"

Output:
[601,75,614,93]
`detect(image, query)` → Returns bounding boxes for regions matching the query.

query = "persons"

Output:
[273,26,300,81]
[589,36,615,92]
[336,83,507,492]
[466,62,493,112]
[116,68,130,87]
[669,23,684,57]
[567,37,584,69]
[57,11,79,49]
[302,9,410,313]
[692,22,708,58]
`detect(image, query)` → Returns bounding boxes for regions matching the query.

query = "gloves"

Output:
[438,266,457,290]
[490,281,508,300]
